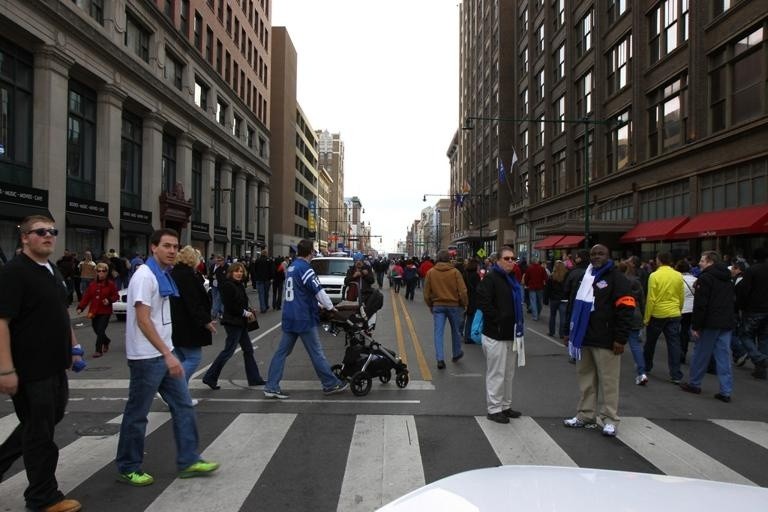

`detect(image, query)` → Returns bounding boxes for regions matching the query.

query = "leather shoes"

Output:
[714,393,730,401]
[249,380,267,386]
[501,408,521,418]
[202,381,221,390]
[679,381,701,393]
[486,412,509,422]
[453,350,463,361]
[438,361,445,369]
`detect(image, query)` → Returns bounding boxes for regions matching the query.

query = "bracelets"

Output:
[2,368,16,375]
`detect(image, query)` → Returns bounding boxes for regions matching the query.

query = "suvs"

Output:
[311,257,354,304]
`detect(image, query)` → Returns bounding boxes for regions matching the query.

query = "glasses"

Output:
[24,227,58,237]
[590,250,606,256]
[216,259,222,262]
[96,268,106,273]
[498,256,519,261]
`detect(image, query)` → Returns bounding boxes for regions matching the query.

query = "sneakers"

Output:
[563,417,598,428]
[602,424,617,435]
[265,391,291,399]
[635,373,648,385]
[323,381,350,396]
[116,470,154,485]
[177,459,219,478]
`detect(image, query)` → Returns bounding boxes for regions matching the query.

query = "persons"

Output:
[392,255,421,267]
[675,260,697,364]
[644,251,685,384]
[153,245,217,407]
[376,256,388,290]
[474,246,522,425]
[56,249,76,309]
[0,214,88,512]
[617,260,648,385]
[117,227,220,487]
[403,260,418,302]
[77,252,95,294]
[203,262,267,389]
[731,260,748,366]
[272,256,285,311]
[392,261,403,293]
[543,260,569,338]
[739,246,768,379]
[206,249,258,273]
[344,253,375,290]
[627,255,648,341]
[564,244,636,438]
[423,249,468,369]
[388,260,396,287]
[420,256,433,287]
[522,256,548,321]
[264,239,349,398]
[98,249,144,278]
[564,248,591,346]
[255,251,272,313]
[76,263,120,358]
[682,251,735,403]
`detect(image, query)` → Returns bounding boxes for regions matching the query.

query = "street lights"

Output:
[319,207,365,254]
[423,194,483,268]
[463,117,589,251]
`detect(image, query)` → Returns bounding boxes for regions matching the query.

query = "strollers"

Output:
[330,281,409,396]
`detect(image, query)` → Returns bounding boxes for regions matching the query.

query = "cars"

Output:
[111,275,211,321]
